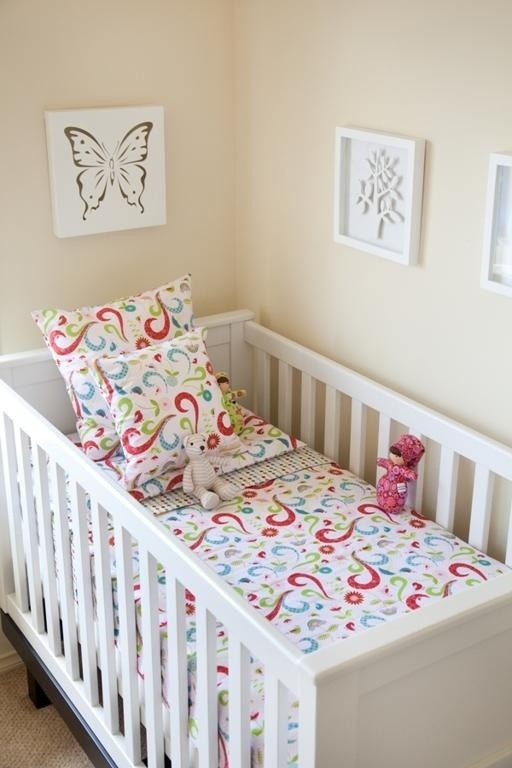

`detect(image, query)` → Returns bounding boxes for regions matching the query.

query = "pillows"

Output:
[30,272,198,466]
[93,325,242,493]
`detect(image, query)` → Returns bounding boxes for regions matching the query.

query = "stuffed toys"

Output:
[182,432,236,510]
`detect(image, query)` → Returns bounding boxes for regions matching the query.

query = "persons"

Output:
[215,370,248,435]
[375,434,426,515]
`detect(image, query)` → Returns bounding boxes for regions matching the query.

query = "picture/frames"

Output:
[481,152,511,297]
[332,125,427,268]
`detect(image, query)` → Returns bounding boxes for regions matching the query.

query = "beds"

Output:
[0,308,511,767]
